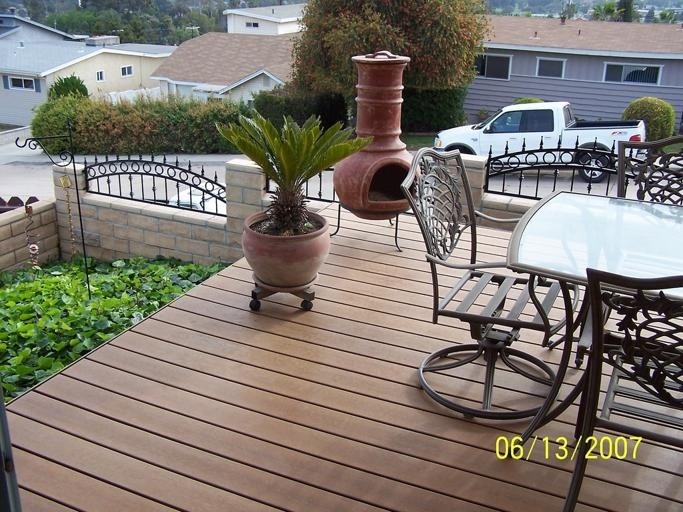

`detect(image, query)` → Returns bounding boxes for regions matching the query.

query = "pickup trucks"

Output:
[433,102,648,183]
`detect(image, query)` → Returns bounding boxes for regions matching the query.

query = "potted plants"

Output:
[215,108,374,288]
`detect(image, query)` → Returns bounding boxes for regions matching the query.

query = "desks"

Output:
[507,189,682,300]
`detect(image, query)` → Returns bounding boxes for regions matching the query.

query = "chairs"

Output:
[403,147,581,421]
[559,266,683,511]
[618,135,683,205]
